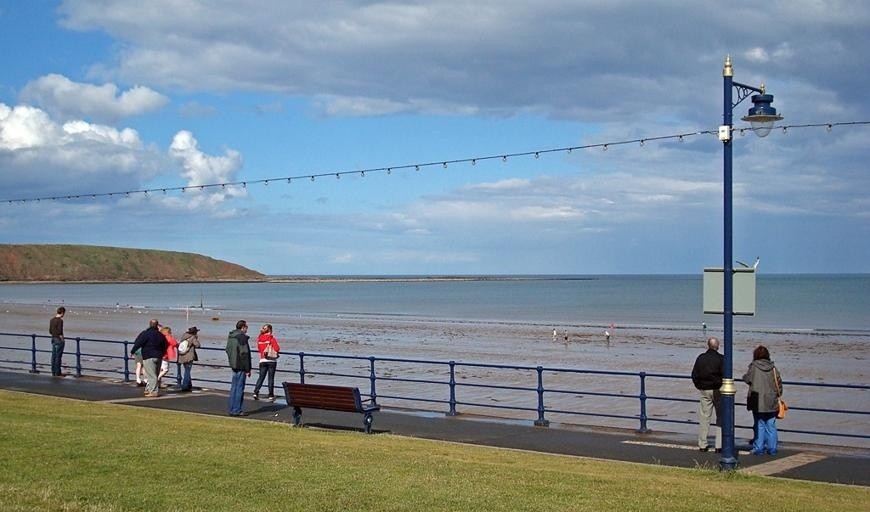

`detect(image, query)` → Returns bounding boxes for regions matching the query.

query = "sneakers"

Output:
[715,449,721,453]
[253,394,258,400]
[752,448,763,455]
[767,451,777,456]
[268,396,276,400]
[700,447,707,452]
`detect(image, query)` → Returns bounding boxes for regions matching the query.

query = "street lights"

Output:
[721,55,783,472]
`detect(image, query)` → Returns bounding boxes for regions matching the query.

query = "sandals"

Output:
[136,381,147,386]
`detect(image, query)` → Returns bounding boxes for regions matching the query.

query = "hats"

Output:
[186,327,200,333]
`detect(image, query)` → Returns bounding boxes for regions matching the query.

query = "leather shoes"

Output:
[240,411,249,416]
[52,373,65,376]
[144,390,159,397]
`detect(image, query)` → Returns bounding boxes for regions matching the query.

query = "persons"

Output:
[177,326,201,391]
[691,336,724,453]
[552,328,558,342]
[48,306,68,377]
[158,327,180,388]
[224,319,252,416]
[252,324,280,401]
[741,344,782,456]
[135,348,149,385]
[702,320,707,331]
[130,319,170,397]
[604,330,610,339]
[563,330,571,344]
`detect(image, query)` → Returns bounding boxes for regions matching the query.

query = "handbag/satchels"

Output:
[194,352,198,361]
[777,400,787,420]
[263,345,279,360]
[713,390,721,408]
[178,339,189,355]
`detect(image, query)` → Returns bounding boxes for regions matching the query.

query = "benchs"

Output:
[282,382,381,434]
